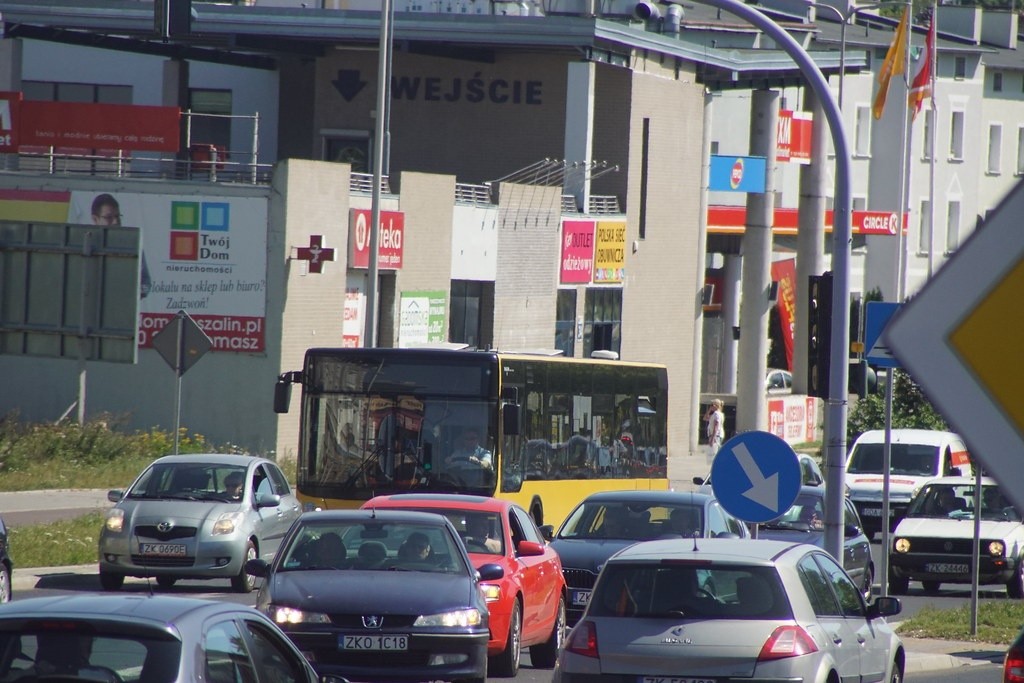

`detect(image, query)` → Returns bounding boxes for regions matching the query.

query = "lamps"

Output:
[482,156,622,189]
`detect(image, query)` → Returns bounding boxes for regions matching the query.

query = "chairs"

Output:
[310,532,347,571]
[602,511,652,536]
[736,577,780,617]
[357,539,389,570]
[169,468,213,492]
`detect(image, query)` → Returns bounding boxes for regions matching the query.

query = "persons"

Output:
[669,509,689,533]
[444,427,493,469]
[91,193,152,299]
[603,508,622,536]
[703,399,724,446]
[941,488,954,510]
[801,506,821,528]
[226,472,242,498]
[407,532,430,562]
[984,488,1009,512]
[462,515,500,552]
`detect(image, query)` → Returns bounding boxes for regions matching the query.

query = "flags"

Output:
[909,14,932,121]
[872,7,909,120]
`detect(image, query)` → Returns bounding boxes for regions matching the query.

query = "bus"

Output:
[272,341,673,545]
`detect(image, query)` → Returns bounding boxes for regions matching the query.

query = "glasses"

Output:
[405,543,428,554]
[225,483,242,489]
[465,436,479,442]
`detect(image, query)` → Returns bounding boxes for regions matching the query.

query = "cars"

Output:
[692,453,876,607]
[843,428,974,543]
[535,489,754,631]
[341,491,570,677]
[889,475,1024,601]
[0,519,16,605]
[243,509,506,683]
[0,594,351,683]
[552,535,907,683]
[766,367,794,397]
[98,453,303,594]
